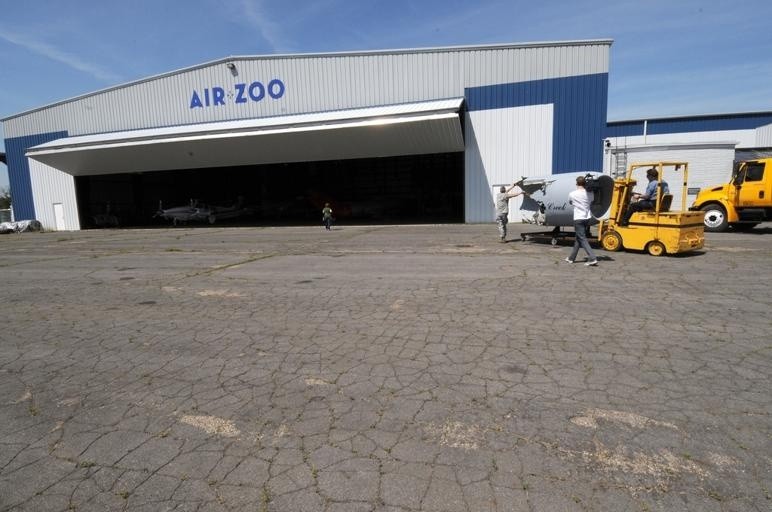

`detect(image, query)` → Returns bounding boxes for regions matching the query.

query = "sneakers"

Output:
[565,257,573,263]
[585,260,598,266]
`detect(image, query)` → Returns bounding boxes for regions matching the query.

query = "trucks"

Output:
[687,157,771,233]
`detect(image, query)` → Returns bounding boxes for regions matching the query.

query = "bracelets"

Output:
[514,183,516,187]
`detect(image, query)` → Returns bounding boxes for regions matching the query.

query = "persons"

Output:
[496,184,526,243]
[565,176,599,266]
[322,203,332,230]
[622,169,670,227]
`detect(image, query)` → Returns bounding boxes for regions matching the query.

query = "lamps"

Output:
[227,64,235,68]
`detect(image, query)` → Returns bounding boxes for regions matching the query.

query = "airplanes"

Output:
[152,194,256,228]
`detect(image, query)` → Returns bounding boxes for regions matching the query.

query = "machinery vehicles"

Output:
[526,160,706,255]
